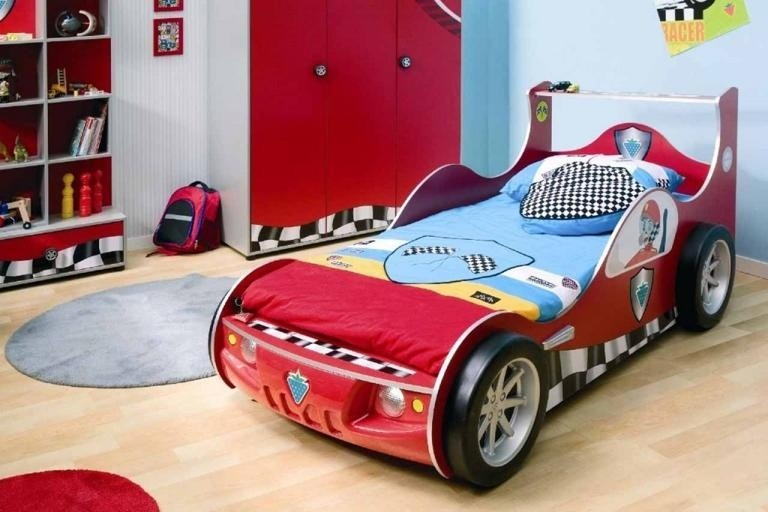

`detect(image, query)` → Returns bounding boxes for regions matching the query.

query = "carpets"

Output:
[4,272,244,389]
[0,467,162,512]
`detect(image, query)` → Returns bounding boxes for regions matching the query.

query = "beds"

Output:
[207,79,740,492]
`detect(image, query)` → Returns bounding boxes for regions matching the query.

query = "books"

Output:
[71,116,104,157]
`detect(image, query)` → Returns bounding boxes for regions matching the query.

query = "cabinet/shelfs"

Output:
[206,0,462,261]
[5,2,125,290]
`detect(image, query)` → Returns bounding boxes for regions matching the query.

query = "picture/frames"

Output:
[151,1,186,57]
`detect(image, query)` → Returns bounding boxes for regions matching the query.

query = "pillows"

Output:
[499,151,687,238]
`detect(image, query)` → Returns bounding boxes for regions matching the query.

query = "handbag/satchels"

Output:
[153,181,221,254]
